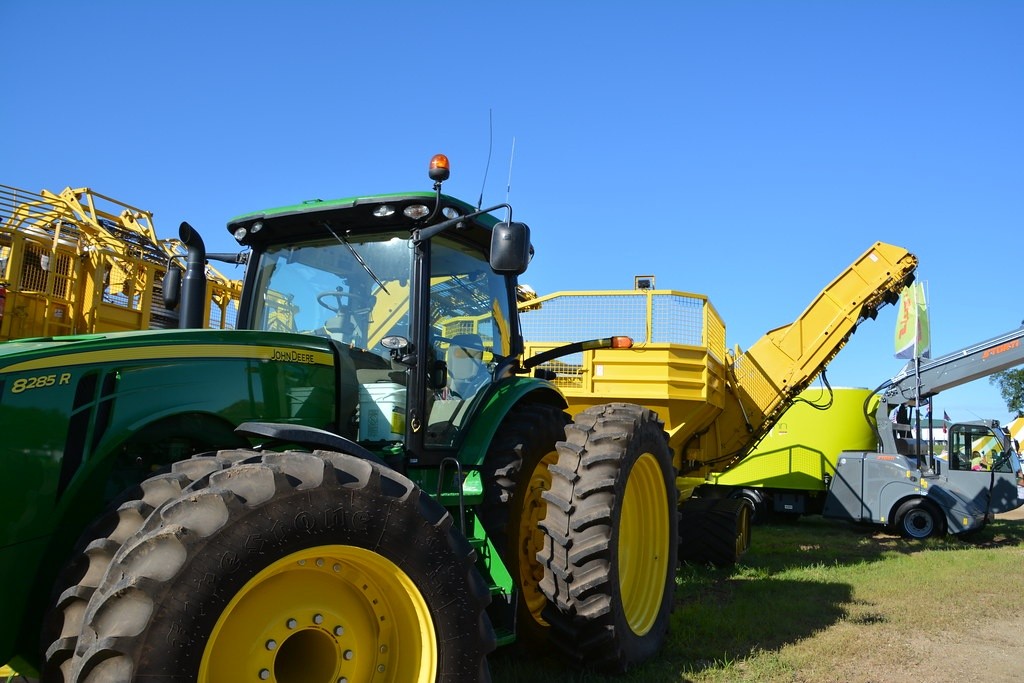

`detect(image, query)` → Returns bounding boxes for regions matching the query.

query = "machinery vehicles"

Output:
[0,151,1023,683]
